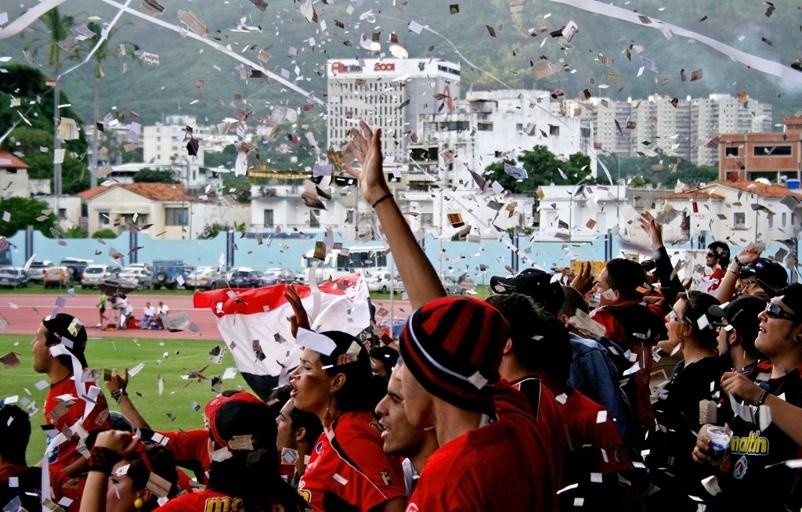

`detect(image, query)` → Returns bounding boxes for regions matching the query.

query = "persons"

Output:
[1,120,802,511]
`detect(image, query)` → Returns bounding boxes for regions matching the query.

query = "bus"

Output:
[302,244,394,295]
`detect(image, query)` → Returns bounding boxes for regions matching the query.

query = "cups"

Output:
[706,427,735,466]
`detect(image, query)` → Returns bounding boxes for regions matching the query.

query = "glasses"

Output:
[707,251,718,258]
[765,300,802,325]
[747,262,766,274]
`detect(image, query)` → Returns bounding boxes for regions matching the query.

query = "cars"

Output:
[392,271,464,297]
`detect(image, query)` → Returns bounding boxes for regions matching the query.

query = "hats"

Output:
[42,312,89,369]
[708,294,767,336]
[737,262,788,290]
[204,390,271,454]
[397,295,511,416]
[756,278,802,317]
